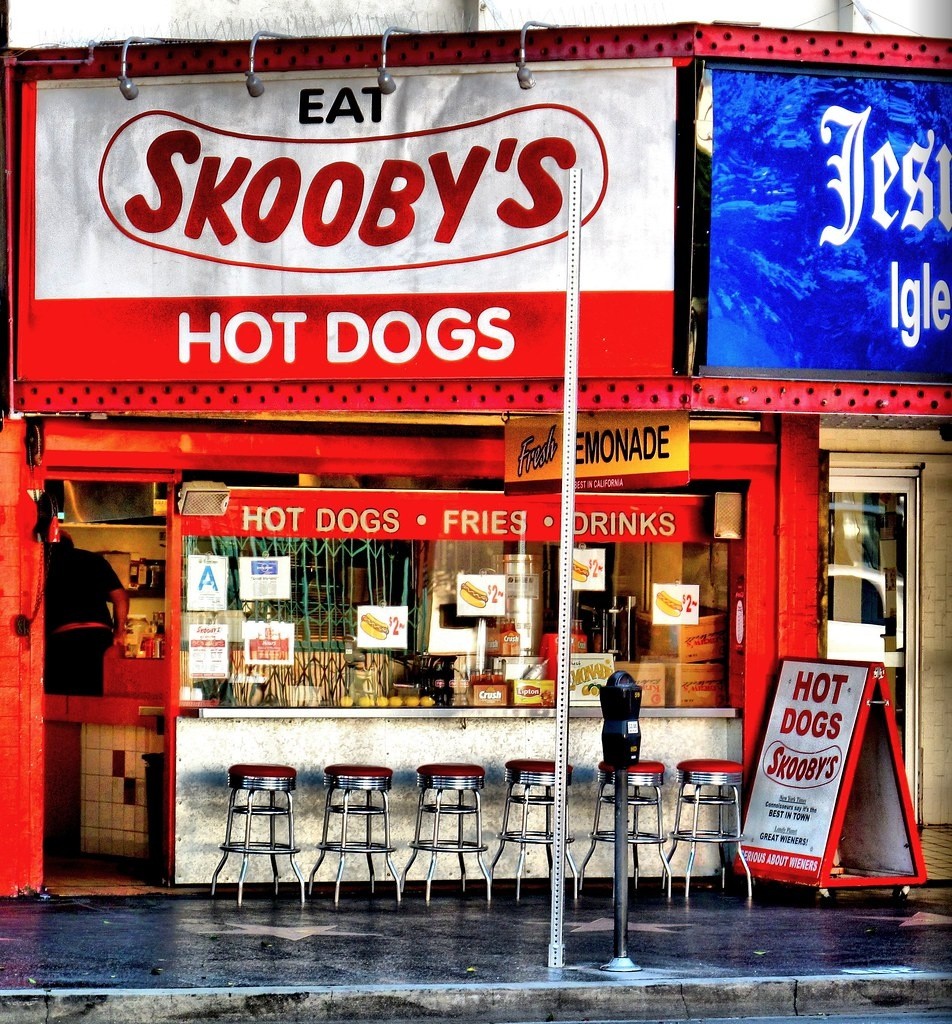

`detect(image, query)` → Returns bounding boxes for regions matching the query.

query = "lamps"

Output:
[117,36,163,101]
[516,20,555,90]
[175,480,231,518]
[245,31,295,98]
[376,26,420,95]
[709,491,747,542]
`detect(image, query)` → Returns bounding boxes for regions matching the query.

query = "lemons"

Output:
[339,695,436,708]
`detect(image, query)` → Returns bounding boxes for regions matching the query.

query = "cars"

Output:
[826,564,903,628]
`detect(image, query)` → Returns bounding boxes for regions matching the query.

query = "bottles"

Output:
[469,668,504,686]
[572,619,601,653]
[412,652,476,706]
[487,617,521,656]
[124,610,165,658]
[130,557,145,589]
[524,658,549,680]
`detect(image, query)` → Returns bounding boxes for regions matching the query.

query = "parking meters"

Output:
[599,672,643,973]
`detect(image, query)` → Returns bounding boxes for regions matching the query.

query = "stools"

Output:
[400,762,491,905]
[487,760,578,904]
[578,760,672,903]
[308,765,402,908]
[211,765,306,907]
[659,760,753,910]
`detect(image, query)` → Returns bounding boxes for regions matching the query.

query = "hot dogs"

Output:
[572,561,590,583]
[656,590,684,617]
[361,613,389,640]
[460,581,488,608]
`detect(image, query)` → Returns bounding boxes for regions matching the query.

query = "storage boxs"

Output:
[507,679,555,707]
[636,611,726,663]
[469,675,507,706]
[666,663,727,708]
[615,661,665,707]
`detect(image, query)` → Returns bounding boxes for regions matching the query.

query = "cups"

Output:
[179,686,190,700]
[191,687,203,700]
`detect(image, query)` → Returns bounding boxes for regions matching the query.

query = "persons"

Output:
[45,530,131,697]
